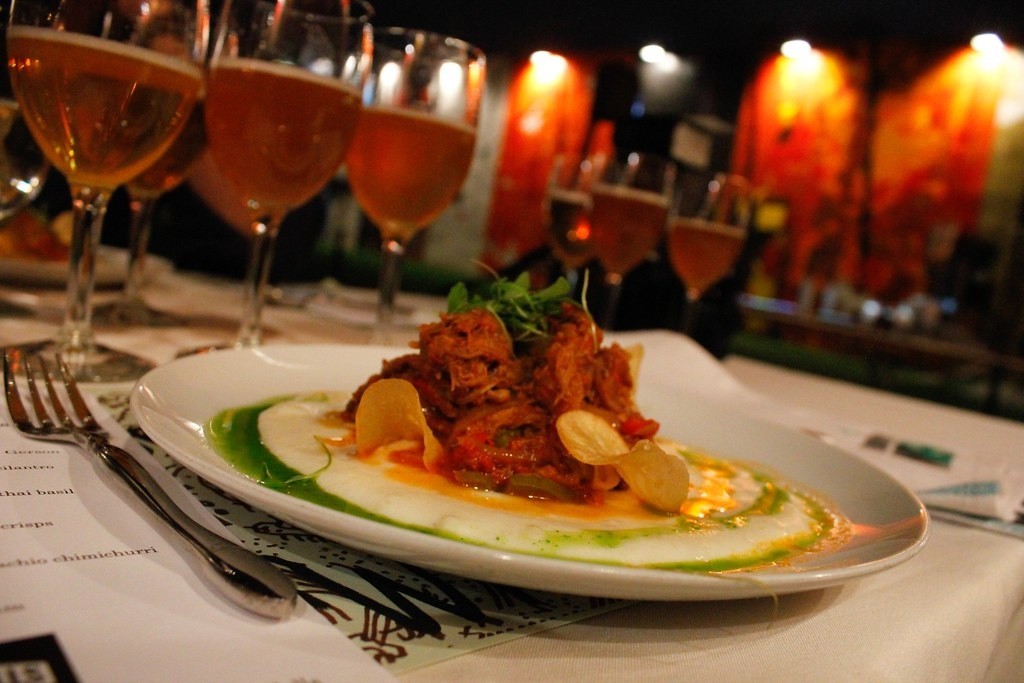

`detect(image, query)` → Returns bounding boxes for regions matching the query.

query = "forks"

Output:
[1,350,301,603]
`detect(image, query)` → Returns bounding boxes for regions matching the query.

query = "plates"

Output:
[0,246,168,283]
[128,329,928,603]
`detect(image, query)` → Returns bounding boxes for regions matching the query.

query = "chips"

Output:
[555,410,689,515]
[354,377,442,471]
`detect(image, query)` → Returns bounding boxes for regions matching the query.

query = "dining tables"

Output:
[0,276,1024,683]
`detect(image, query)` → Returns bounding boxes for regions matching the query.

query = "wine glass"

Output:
[0,1,209,387]
[184,0,374,356]
[44,0,209,330]
[345,23,486,344]
[1,103,49,318]
[589,149,676,325]
[544,155,604,287]
[663,168,750,332]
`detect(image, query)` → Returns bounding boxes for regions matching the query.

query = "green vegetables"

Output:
[442,257,598,349]
